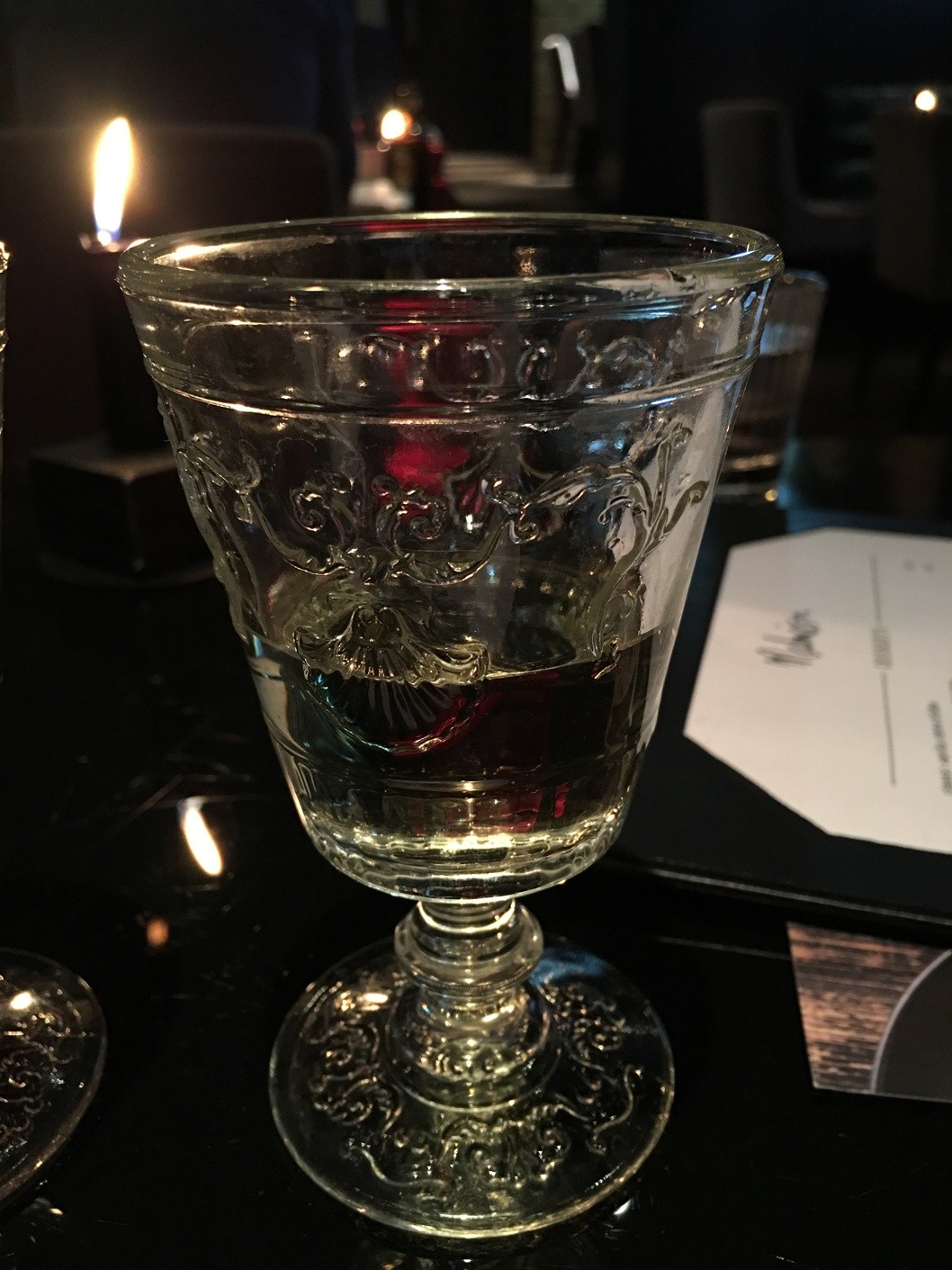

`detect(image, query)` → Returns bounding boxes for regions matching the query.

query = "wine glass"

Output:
[116,211,786,1239]
[0,948,112,1203]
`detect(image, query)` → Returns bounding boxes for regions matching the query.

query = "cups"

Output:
[710,271,825,500]
